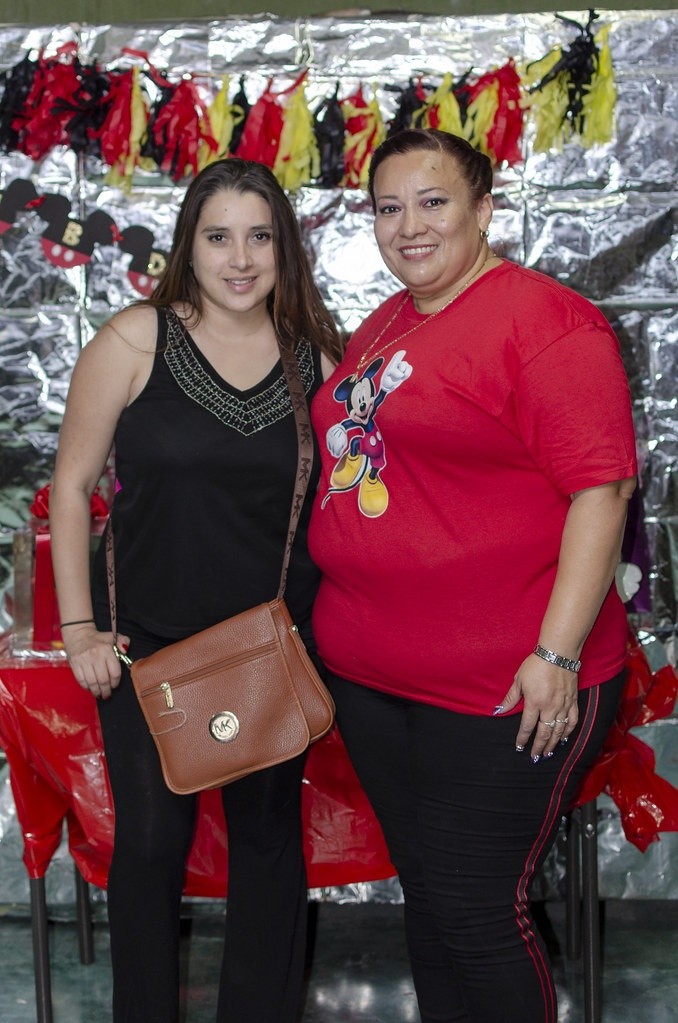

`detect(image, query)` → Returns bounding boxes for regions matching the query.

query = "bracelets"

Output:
[59,619,96,628]
[532,643,582,673]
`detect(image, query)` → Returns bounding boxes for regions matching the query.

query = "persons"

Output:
[47,157,349,1023]
[309,126,637,1023]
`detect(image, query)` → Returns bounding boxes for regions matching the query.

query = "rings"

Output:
[539,719,555,726]
[555,717,569,723]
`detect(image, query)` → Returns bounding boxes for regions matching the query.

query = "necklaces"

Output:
[349,249,488,383]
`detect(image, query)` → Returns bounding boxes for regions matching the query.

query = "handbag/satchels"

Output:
[128,599,337,795]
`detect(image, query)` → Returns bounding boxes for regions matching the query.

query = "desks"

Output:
[0,623,678,1023]
